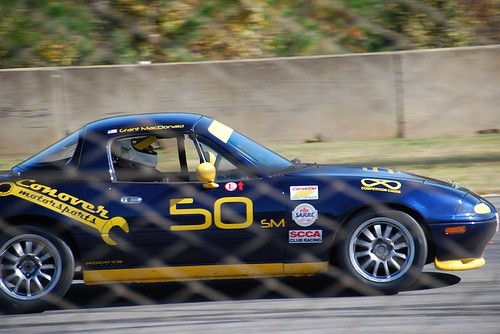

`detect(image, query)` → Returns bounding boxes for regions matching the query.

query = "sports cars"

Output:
[0,112,497,314]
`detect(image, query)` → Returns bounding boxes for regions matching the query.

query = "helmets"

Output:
[110,136,158,168]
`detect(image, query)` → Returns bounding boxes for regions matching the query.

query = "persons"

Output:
[114,136,171,183]
